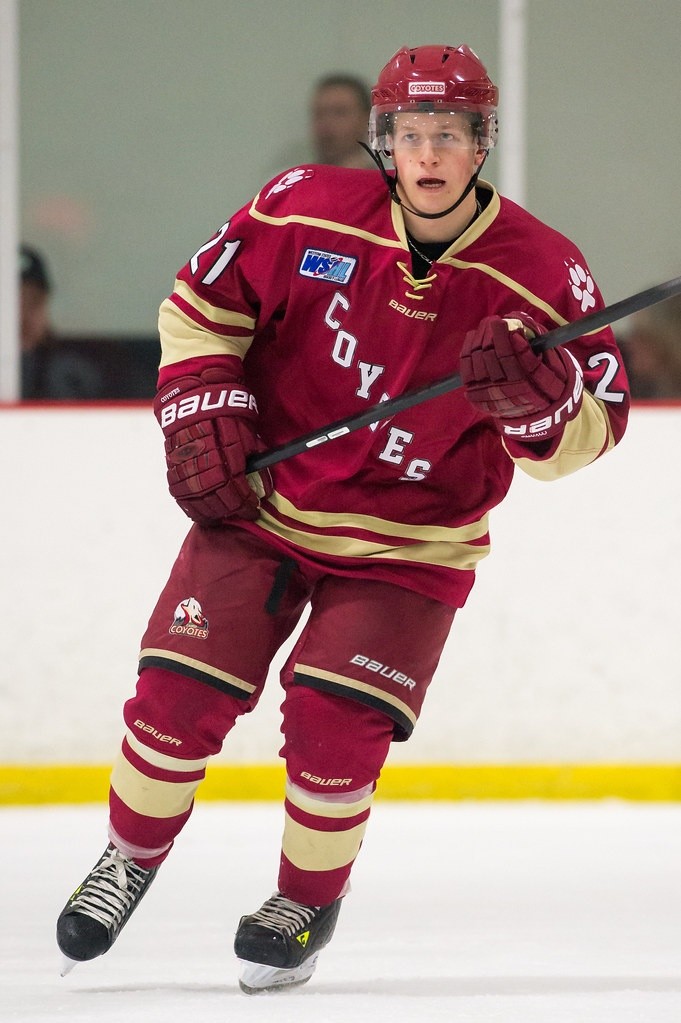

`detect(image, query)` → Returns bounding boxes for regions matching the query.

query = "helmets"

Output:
[370,42,499,159]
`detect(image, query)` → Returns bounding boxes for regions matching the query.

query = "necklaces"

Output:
[409,192,480,273]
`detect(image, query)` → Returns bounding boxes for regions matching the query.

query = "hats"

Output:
[19,247,47,288]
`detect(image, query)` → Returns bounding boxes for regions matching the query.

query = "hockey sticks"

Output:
[245,275,681,473]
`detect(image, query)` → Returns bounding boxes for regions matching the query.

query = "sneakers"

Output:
[47,841,161,986]
[234,893,343,992]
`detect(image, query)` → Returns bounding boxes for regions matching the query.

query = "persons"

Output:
[300,72,381,171]
[50,44,631,993]
[19,247,111,402]
[615,286,681,406]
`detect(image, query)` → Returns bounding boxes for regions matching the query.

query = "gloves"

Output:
[153,367,275,527]
[460,311,584,442]
